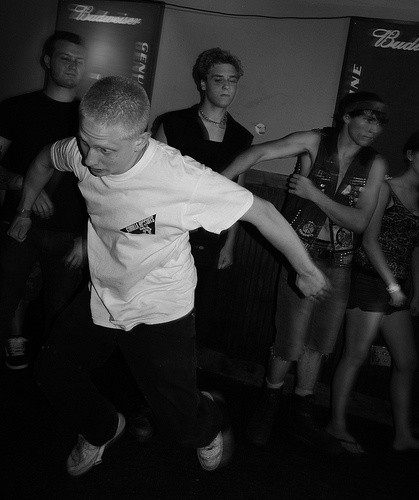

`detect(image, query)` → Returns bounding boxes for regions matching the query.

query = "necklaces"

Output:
[199,105,228,129]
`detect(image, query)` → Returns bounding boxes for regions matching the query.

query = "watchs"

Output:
[14,208,32,216]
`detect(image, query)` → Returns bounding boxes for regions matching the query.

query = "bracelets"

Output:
[13,175,23,183]
[386,283,401,294]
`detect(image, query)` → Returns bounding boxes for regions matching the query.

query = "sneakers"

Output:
[2,336,29,370]
[196,391,223,471]
[67,413,125,475]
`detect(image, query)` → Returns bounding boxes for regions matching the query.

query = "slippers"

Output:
[335,436,363,457]
[391,448,419,455]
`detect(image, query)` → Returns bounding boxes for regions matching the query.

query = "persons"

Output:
[154,46,254,371]
[0,30,91,370]
[6,76,331,477]
[324,130,419,458]
[219,91,392,452]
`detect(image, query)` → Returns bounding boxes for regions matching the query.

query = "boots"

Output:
[291,391,315,443]
[246,381,283,444]
